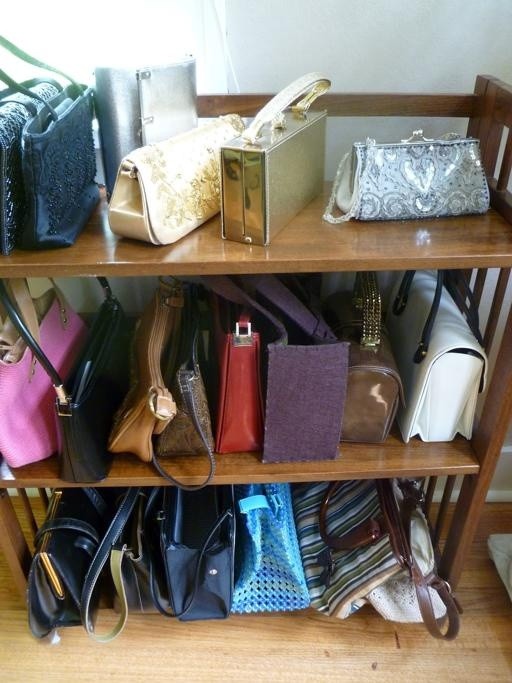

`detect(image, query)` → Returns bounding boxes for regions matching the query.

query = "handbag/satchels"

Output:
[1,34,101,251]
[220,71,333,247]
[0,76,59,257]
[96,60,200,201]
[322,128,490,223]
[1,268,488,491]
[27,477,464,641]
[107,111,244,247]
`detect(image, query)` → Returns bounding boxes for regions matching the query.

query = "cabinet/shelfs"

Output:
[1,77,512,613]
